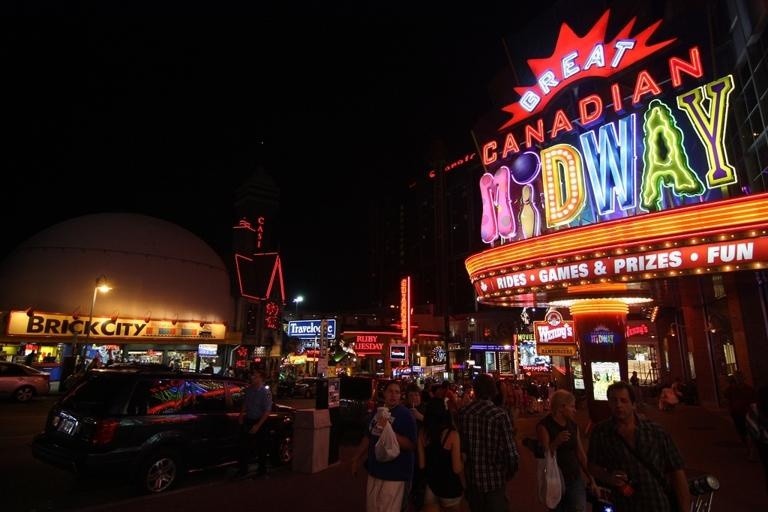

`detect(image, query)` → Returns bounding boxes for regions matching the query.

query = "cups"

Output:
[378,407,390,429]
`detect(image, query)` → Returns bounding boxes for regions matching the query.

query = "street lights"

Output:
[81,274,114,365]
[292,295,304,320]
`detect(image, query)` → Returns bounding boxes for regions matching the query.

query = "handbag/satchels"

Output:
[352,436,375,465]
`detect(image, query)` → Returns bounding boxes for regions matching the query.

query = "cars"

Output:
[31,368,296,494]
[295,376,320,399]
[1,361,51,402]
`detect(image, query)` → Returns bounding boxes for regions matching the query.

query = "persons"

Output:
[524,374,549,403]
[368,374,518,510]
[538,374,689,509]
[238,369,271,480]
[25,349,235,377]
